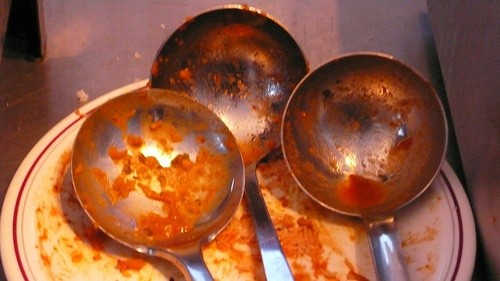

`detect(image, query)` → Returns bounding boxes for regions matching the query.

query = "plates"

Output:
[0,77,479,281]
